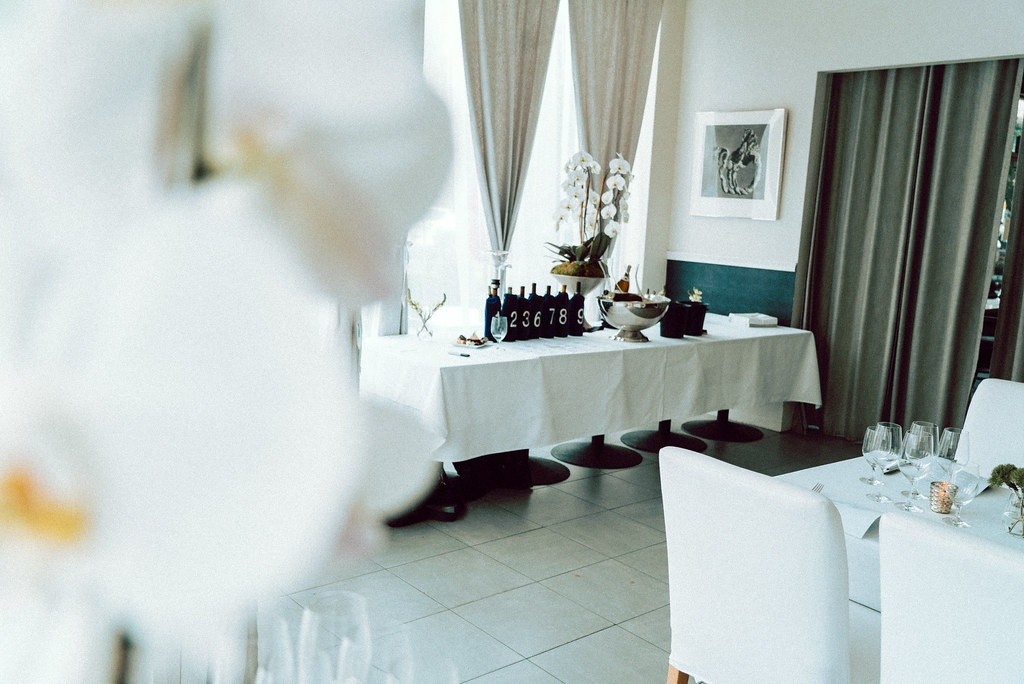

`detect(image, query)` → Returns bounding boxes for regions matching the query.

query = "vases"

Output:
[550,271,610,329]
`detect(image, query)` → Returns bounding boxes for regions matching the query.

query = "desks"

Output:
[357,312,821,527]
[771,449,1024,614]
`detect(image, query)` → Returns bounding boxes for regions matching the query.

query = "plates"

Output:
[451,341,486,348]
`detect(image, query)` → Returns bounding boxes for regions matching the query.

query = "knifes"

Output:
[449,351,468,357]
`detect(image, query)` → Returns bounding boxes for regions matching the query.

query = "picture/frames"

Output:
[690,108,789,221]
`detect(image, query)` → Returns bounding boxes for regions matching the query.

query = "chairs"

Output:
[659,445,882,684]
[878,511,1024,684]
[953,378,1024,493]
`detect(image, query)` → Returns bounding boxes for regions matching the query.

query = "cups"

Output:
[1008,490,1024,519]
[660,300,707,339]
[930,481,959,514]
[865,422,902,504]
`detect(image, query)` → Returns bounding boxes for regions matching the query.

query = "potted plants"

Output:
[987,463,1024,536]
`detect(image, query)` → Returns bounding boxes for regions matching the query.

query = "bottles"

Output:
[615,265,631,293]
[486,282,585,342]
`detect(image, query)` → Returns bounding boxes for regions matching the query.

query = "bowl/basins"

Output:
[597,293,671,341]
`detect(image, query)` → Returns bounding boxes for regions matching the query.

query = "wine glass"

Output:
[212,590,458,684]
[942,460,980,529]
[901,421,939,499]
[895,430,933,513]
[859,426,892,486]
[935,427,970,483]
[491,316,508,352]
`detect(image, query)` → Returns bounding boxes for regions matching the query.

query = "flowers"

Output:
[543,150,638,278]
